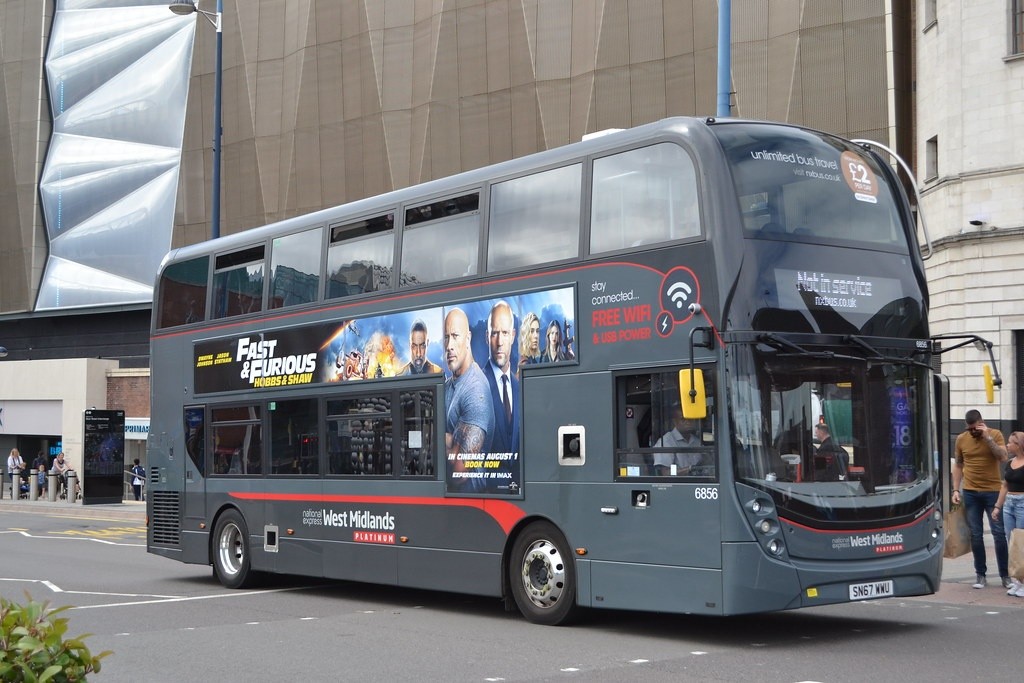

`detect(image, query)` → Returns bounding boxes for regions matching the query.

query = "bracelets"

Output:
[953,489,959,492]
[689,465,692,472]
[994,506,1001,510]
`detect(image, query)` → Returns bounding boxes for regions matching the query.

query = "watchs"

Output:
[986,436,992,443]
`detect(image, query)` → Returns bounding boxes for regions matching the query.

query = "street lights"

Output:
[168,1,223,241]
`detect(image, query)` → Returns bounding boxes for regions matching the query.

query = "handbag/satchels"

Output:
[944,503,971,559]
[1008,528,1023,578]
[133,477,144,485]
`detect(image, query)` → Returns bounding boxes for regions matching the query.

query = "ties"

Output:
[501,374,511,430]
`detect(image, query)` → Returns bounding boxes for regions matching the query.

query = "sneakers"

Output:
[1015,584,1024,597]
[1007,583,1023,595]
[1002,577,1014,588]
[973,575,986,588]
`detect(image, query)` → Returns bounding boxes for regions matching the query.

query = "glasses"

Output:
[966,428,978,431]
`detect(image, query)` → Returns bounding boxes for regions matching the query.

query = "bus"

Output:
[144,115,1003,627]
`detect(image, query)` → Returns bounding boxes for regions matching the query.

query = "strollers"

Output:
[60,469,81,500]
[15,468,30,499]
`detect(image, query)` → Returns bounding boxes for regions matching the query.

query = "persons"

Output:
[130,459,146,501]
[393,300,576,492]
[811,423,849,472]
[8,448,81,500]
[652,405,711,478]
[934,410,1024,598]
